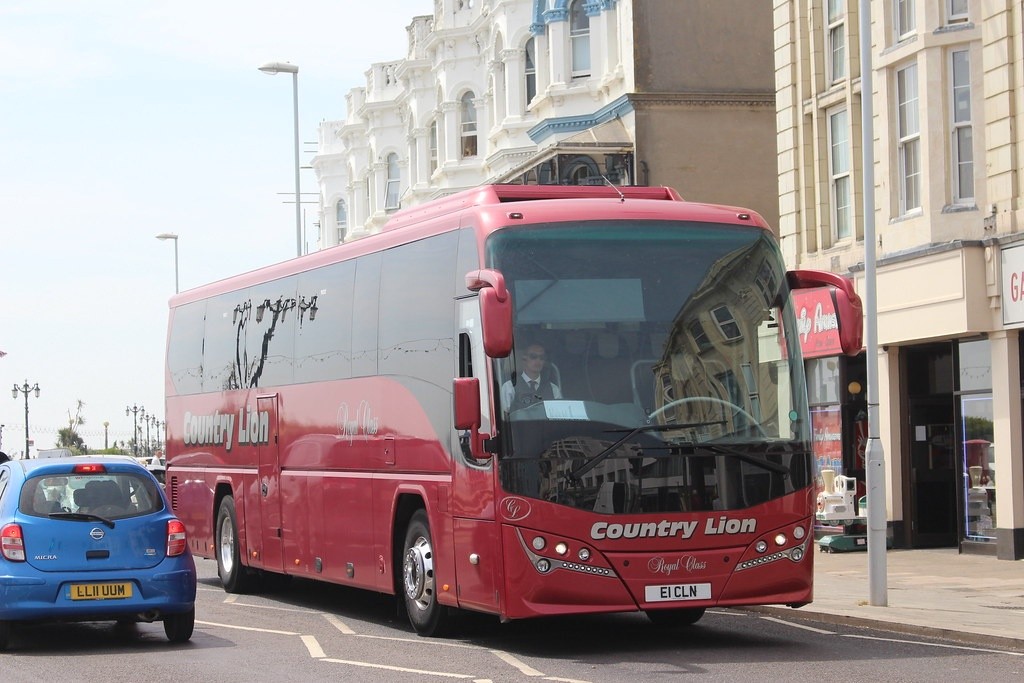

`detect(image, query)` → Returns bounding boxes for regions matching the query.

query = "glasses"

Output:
[524,353,545,360]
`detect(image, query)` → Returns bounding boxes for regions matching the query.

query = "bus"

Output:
[164,185,865,639]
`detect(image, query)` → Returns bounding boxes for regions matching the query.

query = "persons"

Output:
[150,448,165,466]
[499,341,564,417]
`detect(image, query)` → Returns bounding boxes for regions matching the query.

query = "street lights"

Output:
[157,232,179,292]
[11,378,40,459]
[103,420,109,450]
[139,412,156,458]
[259,61,301,256]
[150,418,165,451]
[125,403,145,458]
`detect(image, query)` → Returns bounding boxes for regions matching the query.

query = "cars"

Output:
[0,457,197,645]
[38,454,166,514]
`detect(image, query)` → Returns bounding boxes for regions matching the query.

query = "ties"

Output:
[528,380,536,391]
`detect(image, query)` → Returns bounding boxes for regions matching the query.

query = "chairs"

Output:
[74,480,128,514]
[502,357,562,392]
